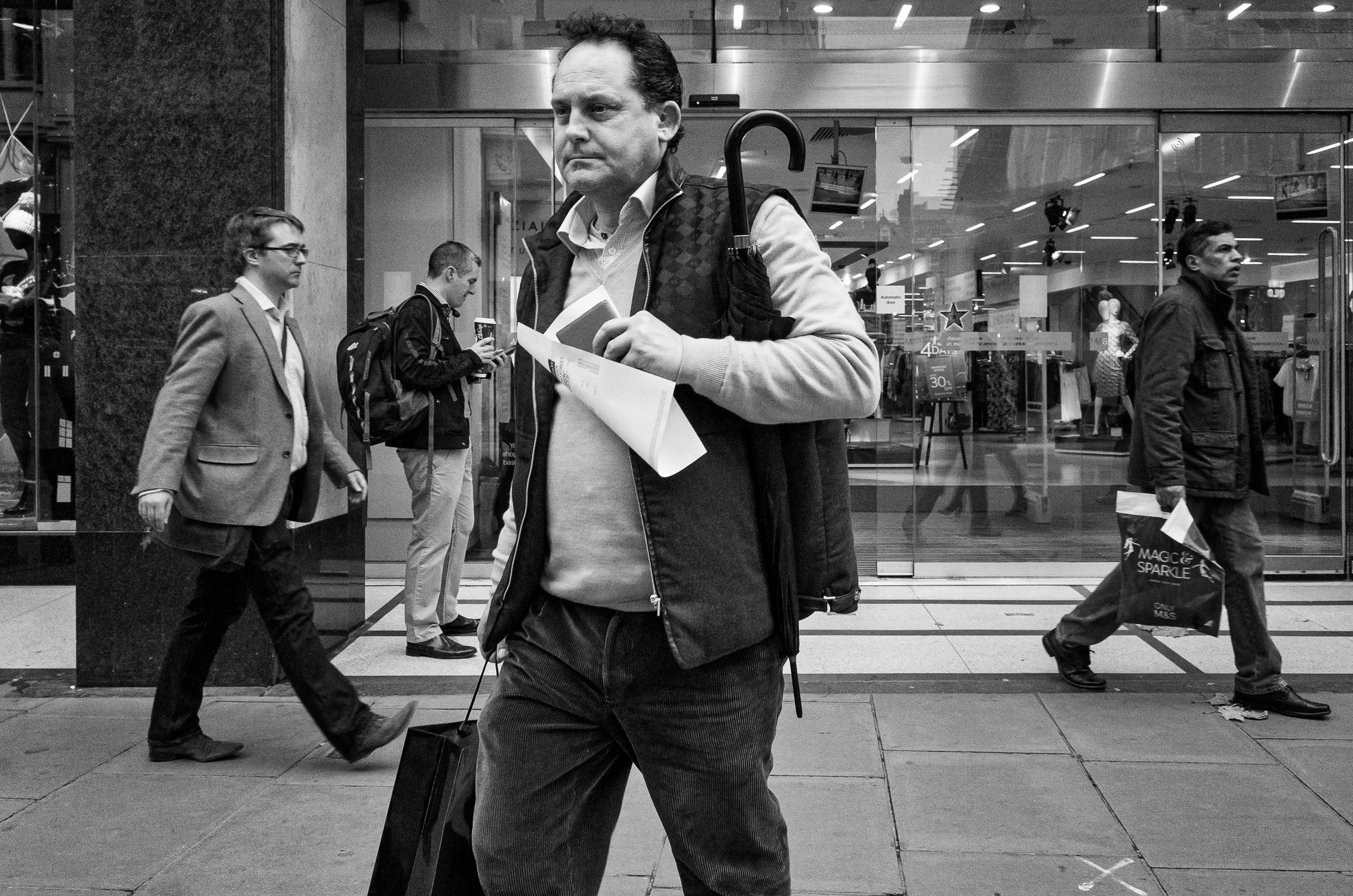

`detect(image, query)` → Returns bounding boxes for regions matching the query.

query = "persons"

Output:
[865,258,881,292]
[384,240,507,658]
[470,11,882,895]
[130,207,419,764]
[1042,221,1333,718]
[901,328,1032,544]
[0,190,67,516]
[1092,299,1139,435]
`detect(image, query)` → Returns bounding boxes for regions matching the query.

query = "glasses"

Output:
[245,248,309,261]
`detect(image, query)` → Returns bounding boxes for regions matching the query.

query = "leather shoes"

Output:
[439,614,482,636]
[1043,628,1108,689]
[1232,681,1333,716]
[406,633,477,660]
[4,485,38,514]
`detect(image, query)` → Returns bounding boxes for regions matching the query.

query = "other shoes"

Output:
[344,699,415,764]
[967,527,1002,537]
[148,730,245,762]
[901,516,919,543]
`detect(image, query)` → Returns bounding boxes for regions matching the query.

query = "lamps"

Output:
[1040,197,1198,272]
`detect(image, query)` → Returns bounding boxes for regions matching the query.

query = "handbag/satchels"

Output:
[366,646,500,896]
[1115,490,1225,639]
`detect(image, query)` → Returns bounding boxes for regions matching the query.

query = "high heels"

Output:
[937,494,963,518]
[1006,493,1028,517]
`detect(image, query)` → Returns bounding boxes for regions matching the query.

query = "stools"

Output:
[916,397,969,472]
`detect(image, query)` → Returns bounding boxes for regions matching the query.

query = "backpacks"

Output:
[336,292,444,446]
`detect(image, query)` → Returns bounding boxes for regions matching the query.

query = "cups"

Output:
[472,317,497,377]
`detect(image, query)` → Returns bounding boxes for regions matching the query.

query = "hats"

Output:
[4,192,41,240]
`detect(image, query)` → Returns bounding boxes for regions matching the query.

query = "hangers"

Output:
[1297,348,1311,358]
